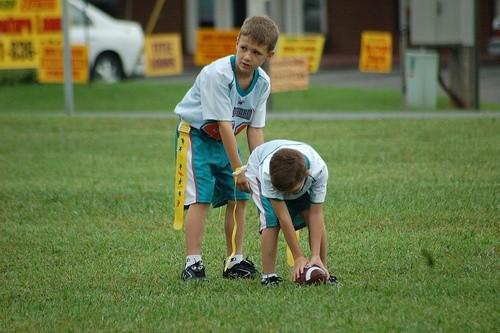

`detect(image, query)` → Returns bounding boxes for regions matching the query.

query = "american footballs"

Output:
[294,266,327,288]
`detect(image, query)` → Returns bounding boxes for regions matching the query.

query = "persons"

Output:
[245,139,338,288]
[174,15,279,282]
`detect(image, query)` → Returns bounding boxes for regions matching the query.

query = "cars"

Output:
[67,0,146,85]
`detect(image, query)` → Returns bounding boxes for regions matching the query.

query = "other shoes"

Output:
[223,257,257,280]
[328,274,338,283]
[261,275,284,288]
[181,261,206,281]
